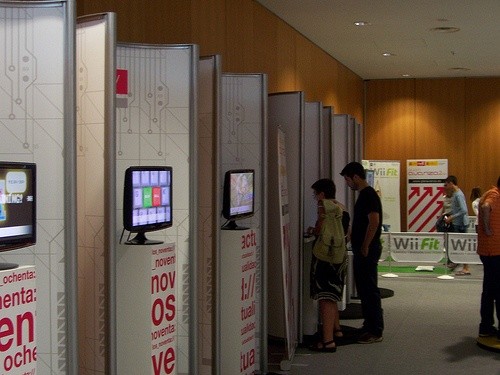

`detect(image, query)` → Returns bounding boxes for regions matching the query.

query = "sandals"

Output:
[321,330,346,341]
[308,341,336,352]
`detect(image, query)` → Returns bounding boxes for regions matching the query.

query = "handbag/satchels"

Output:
[436,214,455,232]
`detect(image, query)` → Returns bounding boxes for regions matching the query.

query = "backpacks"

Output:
[312,212,345,263]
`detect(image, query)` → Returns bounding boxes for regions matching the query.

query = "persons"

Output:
[476,176,500,349]
[470,187,483,235]
[308,178,350,353]
[340,161,384,344]
[435,175,472,275]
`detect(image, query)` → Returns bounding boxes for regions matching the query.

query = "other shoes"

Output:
[455,271,472,276]
[349,327,385,344]
[479,326,498,337]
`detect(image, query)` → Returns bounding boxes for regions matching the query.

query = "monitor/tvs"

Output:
[221,169,255,230]
[123,165,173,246]
[0,162,37,269]
